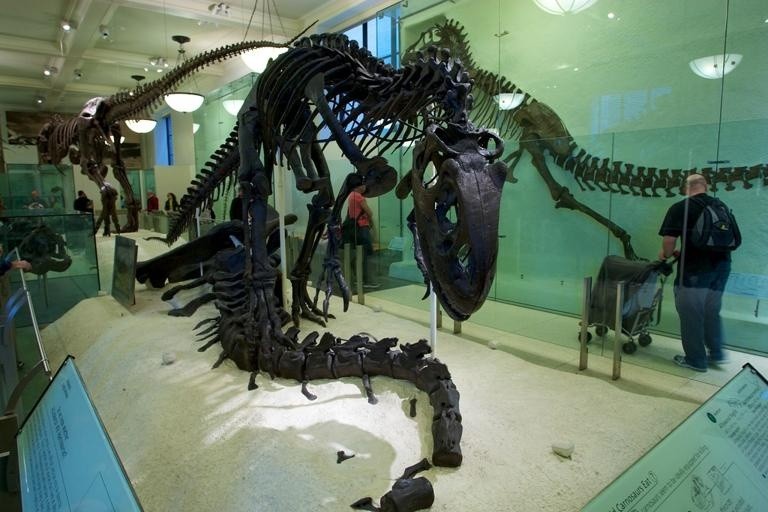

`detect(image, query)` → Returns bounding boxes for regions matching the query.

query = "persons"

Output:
[229,188,252,224]
[163,192,179,212]
[655,172,743,371]
[23,190,48,209]
[73,190,94,213]
[143,191,160,213]
[347,185,381,289]
[0,257,32,277]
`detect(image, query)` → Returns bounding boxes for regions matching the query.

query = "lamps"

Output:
[532,0,605,17]
[686,51,746,79]
[125,74,158,133]
[496,27,523,110]
[164,31,205,113]
[237,0,293,75]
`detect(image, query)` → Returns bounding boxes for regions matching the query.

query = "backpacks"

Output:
[341,205,366,244]
[688,196,743,252]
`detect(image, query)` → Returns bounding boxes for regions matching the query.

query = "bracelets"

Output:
[663,254,671,260]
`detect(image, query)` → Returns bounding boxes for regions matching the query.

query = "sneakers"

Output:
[352,276,383,289]
[671,354,708,373]
[706,352,731,367]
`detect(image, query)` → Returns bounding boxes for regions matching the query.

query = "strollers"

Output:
[578,247,679,355]
